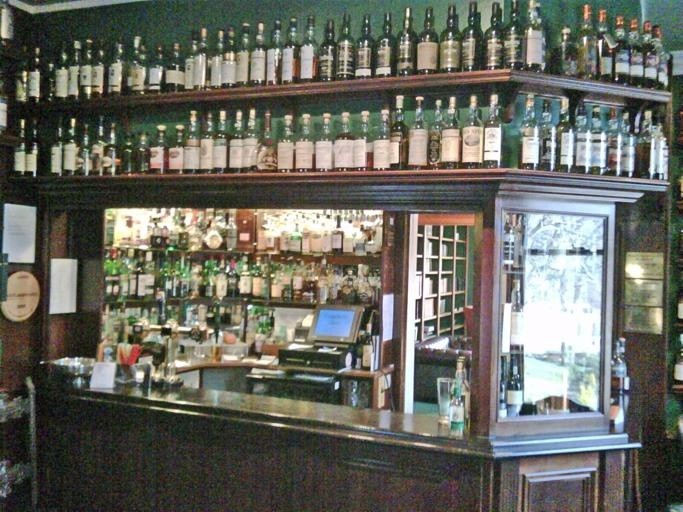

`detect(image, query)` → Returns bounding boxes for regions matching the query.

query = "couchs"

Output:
[415,334,473,405]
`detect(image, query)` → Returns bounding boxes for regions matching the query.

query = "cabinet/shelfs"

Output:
[1,69,672,203]
[71,209,397,410]
[417,225,470,344]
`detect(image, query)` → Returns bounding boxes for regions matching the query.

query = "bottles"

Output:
[450,380,464,434]
[452,360,469,432]
[354,331,365,368]
[558,5,669,90]
[458,356,470,419]
[103,206,238,252]
[104,247,380,305]
[256,208,384,257]
[12,92,504,176]
[519,94,669,182]
[102,299,246,378]
[361,324,373,370]
[500,375,521,417]
[247,302,274,356]
[17,1,547,107]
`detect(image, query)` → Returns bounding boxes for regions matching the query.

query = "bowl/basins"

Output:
[52,357,95,376]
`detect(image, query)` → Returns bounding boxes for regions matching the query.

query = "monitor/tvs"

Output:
[305,304,366,351]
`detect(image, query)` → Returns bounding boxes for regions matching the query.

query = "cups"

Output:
[437,377,455,420]
[185,345,224,364]
